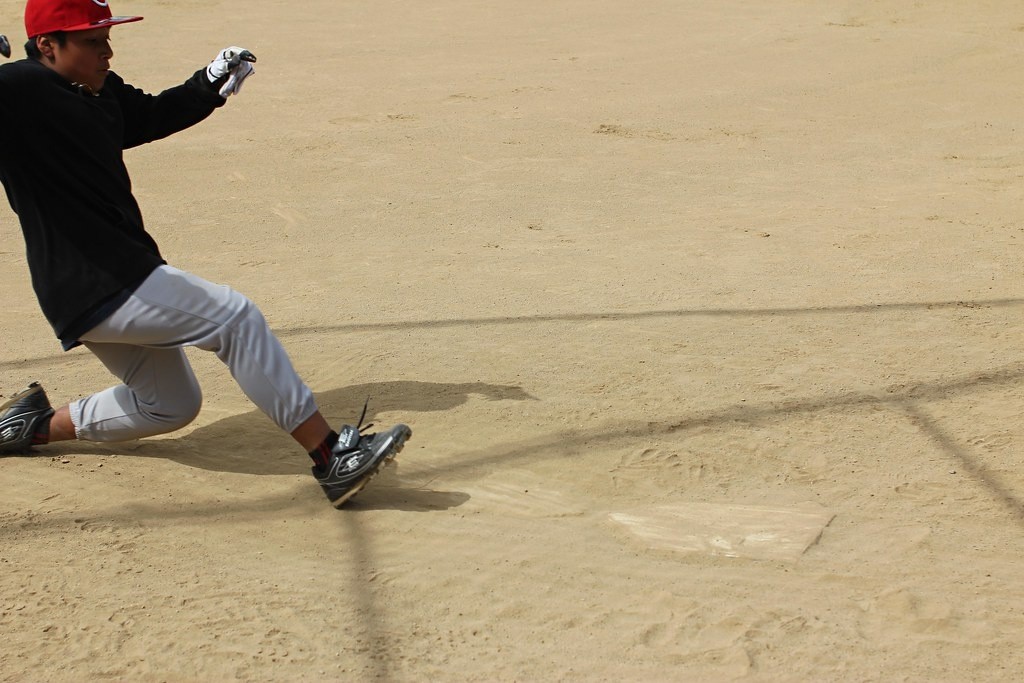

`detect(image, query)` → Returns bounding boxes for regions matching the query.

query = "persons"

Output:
[1,0,413,509]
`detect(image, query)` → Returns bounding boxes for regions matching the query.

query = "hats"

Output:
[24,0,143,39]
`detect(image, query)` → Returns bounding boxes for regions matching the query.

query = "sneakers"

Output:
[0,381,55,454]
[311,395,412,510]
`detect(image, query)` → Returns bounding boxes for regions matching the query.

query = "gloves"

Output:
[0,35,10,58]
[207,46,256,99]
